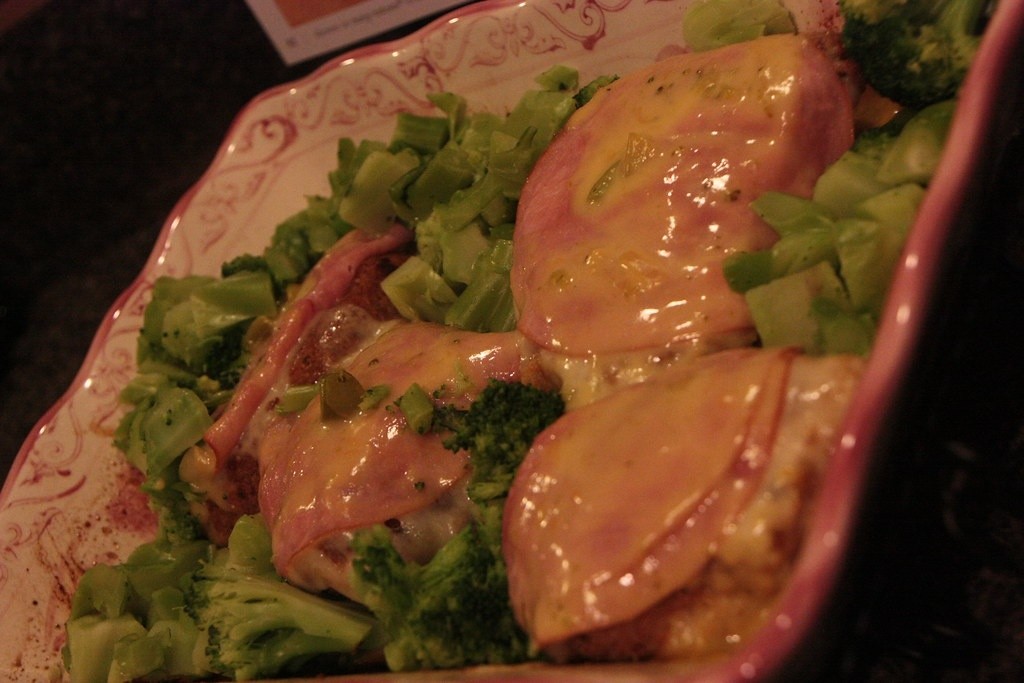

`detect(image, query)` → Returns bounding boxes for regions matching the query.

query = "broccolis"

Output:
[48,0,990,683]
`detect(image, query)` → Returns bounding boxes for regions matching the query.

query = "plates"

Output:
[0,0,1024,683]
